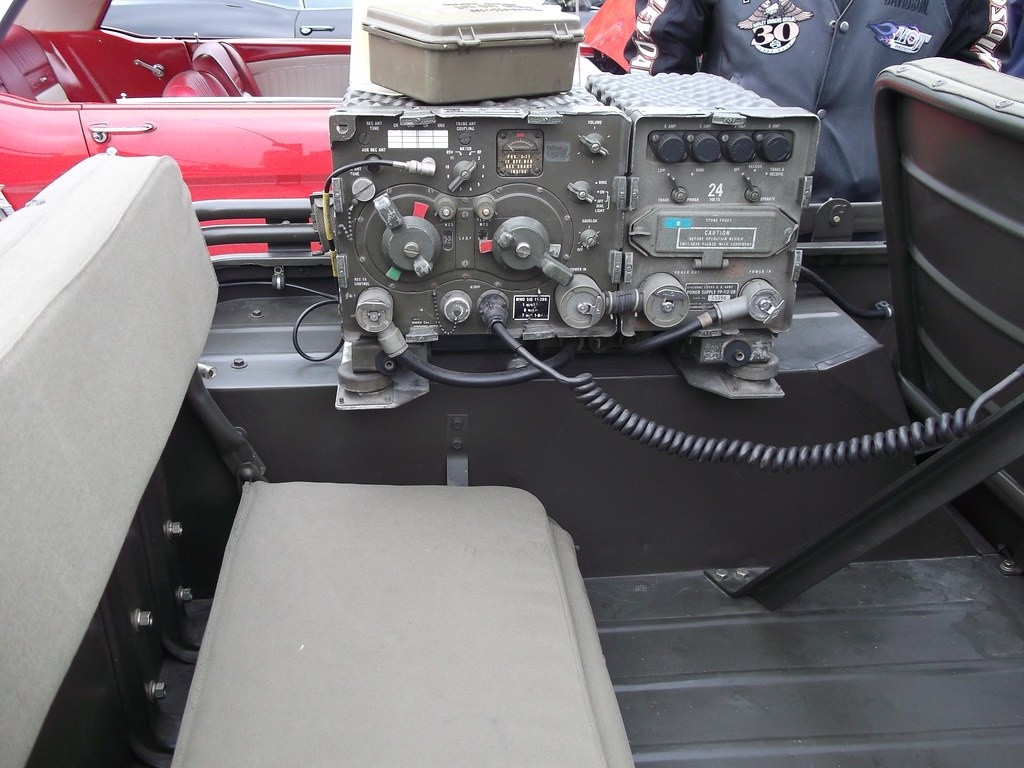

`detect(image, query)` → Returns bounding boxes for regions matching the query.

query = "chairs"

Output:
[162,41,264,97]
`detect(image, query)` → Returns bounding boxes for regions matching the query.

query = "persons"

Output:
[579,1,1024,202]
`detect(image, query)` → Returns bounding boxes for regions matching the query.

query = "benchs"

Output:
[0,152,635,768]
[707,56,1024,594]
[0,24,76,104]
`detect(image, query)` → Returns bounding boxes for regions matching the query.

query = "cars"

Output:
[0,0,598,293]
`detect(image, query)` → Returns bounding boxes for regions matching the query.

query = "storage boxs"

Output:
[362,0,585,105]
